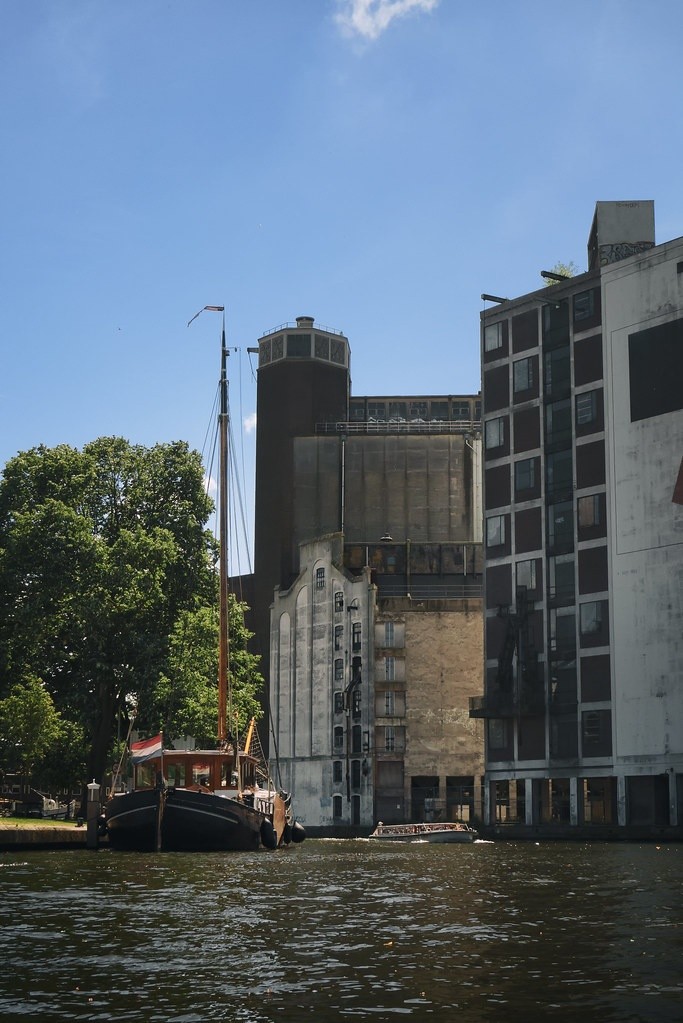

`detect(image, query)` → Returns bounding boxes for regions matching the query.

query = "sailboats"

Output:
[104,301,305,851]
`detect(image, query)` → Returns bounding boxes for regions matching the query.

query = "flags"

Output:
[132,736,162,765]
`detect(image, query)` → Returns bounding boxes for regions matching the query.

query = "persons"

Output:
[373,822,466,836]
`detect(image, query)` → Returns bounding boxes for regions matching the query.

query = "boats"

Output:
[369,822,478,844]
